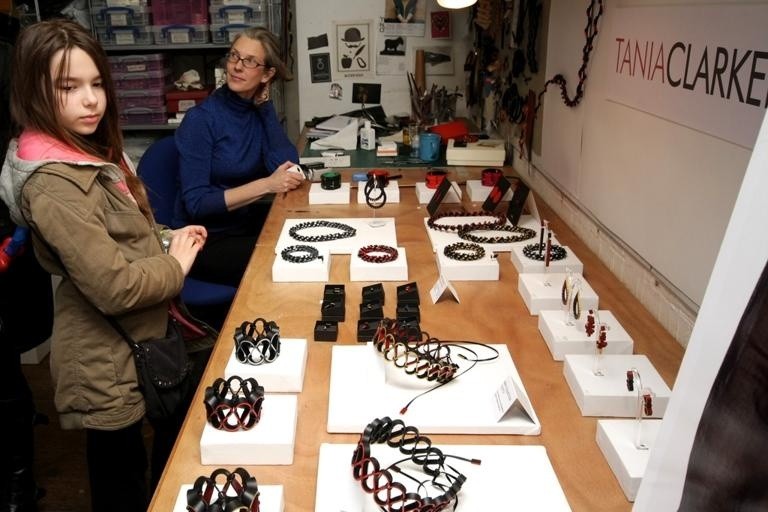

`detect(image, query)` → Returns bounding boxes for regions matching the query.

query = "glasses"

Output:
[225,53,274,69]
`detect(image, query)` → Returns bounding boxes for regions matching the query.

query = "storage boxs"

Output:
[91,1,149,15]
[112,95,164,116]
[110,74,163,96]
[150,0,210,24]
[92,27,154,44]
[152,23,210,45]
[208,3,265,20]
[208,21,265,42]
[90,12,150,25]
[110,53,165,73]
[164,89,211,114]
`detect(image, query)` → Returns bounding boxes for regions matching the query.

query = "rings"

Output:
[318,279,425,344]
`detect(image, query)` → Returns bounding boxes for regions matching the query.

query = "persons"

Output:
[1,18,207,509]
[174,27,304,283]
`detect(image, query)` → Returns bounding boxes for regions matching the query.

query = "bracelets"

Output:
[290,220,356,243]
[424,209,507,231]
[357,246,398,261]
[455,224,537,242]
[441,242,486,261]
[524,244,568,261]
[280,244,319,263]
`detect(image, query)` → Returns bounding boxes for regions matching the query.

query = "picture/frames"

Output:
[430,9,454,41]
[335,23,373,71]
